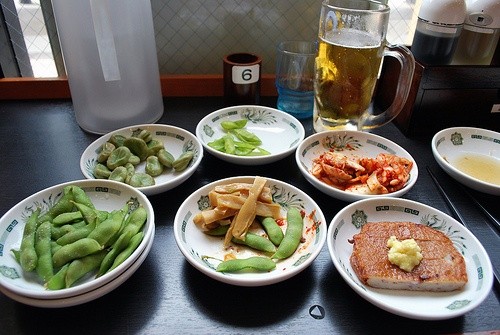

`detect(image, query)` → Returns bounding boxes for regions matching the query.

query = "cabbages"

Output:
[312,152,414,196]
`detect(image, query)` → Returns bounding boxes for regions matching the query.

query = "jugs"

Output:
[50,0,163,134]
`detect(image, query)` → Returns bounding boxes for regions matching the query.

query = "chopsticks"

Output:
[409,140,500,290]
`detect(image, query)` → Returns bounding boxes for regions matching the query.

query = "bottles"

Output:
[451,0,500,66]
[411,0,467,66]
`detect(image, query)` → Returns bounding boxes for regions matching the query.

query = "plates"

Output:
[0,179,156,307]
[79,123,204,196]
[296,130,418,201]
[432,126,500,195]
[196,105,306,164]
[173,176,327,285]
[328,197,494,320]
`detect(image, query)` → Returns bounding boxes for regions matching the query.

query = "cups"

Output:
[312,1,415,132]
[223,52,263,107]
[276,42,320,121]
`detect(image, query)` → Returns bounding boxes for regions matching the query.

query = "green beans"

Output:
[93,130,193,188]
[202,206,303,272]
[10,184,146,289]
